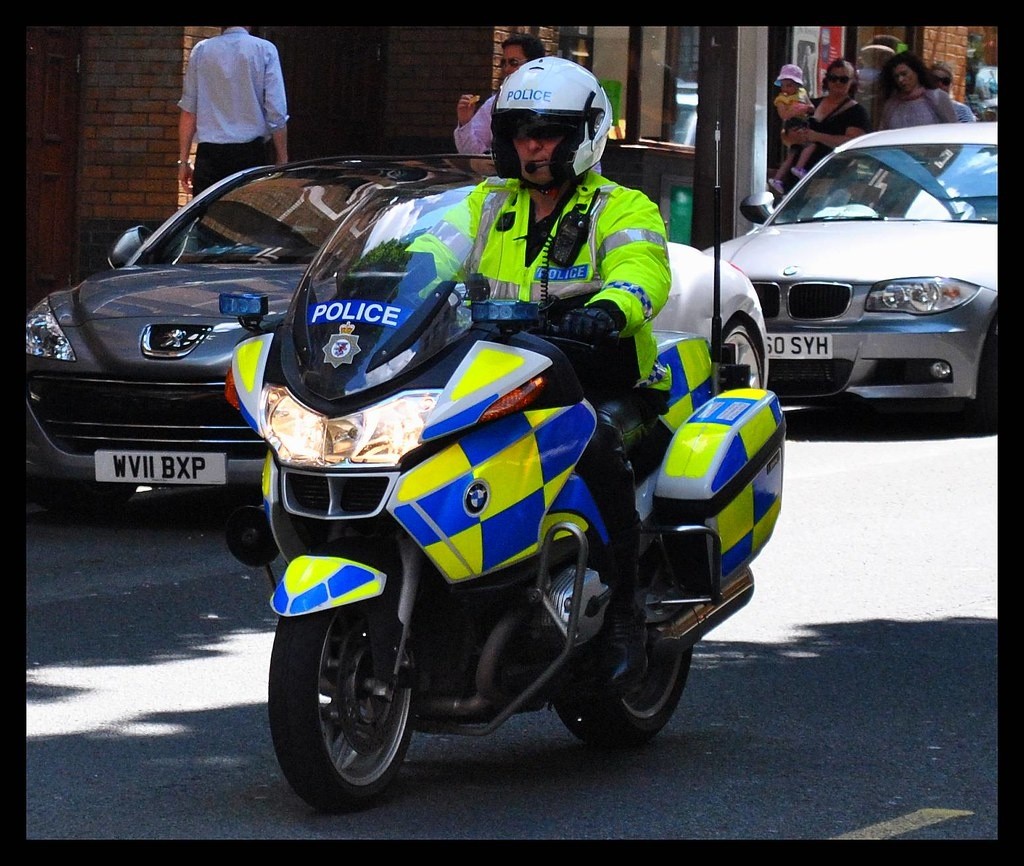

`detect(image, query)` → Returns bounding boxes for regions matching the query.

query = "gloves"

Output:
[559,308,616,339]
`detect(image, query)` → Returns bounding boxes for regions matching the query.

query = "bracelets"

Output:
[179,163,191,167]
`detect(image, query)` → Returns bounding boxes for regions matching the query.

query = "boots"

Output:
[588,510,649,693]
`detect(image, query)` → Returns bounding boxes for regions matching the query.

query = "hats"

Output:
[774,64,804,87]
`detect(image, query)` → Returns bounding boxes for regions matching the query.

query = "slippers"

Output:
[768,178,784,194]
[791,166,808,180]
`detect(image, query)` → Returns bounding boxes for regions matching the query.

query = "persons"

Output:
[403,55,671,697]
[453,33,545,155]
[767,34,977,195]
[178,27,290,292]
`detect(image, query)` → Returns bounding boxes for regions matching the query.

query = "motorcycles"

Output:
[223,173,788,811]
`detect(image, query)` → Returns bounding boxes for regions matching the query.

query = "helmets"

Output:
[491,56,614,183]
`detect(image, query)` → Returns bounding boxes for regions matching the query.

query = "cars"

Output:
[700,119,999,439]
[26,153,504,520]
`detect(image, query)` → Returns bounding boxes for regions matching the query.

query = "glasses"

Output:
[828,74,853,84]
[505,114,579,139]
[931,75,951,86]
[500,58,528,68]
